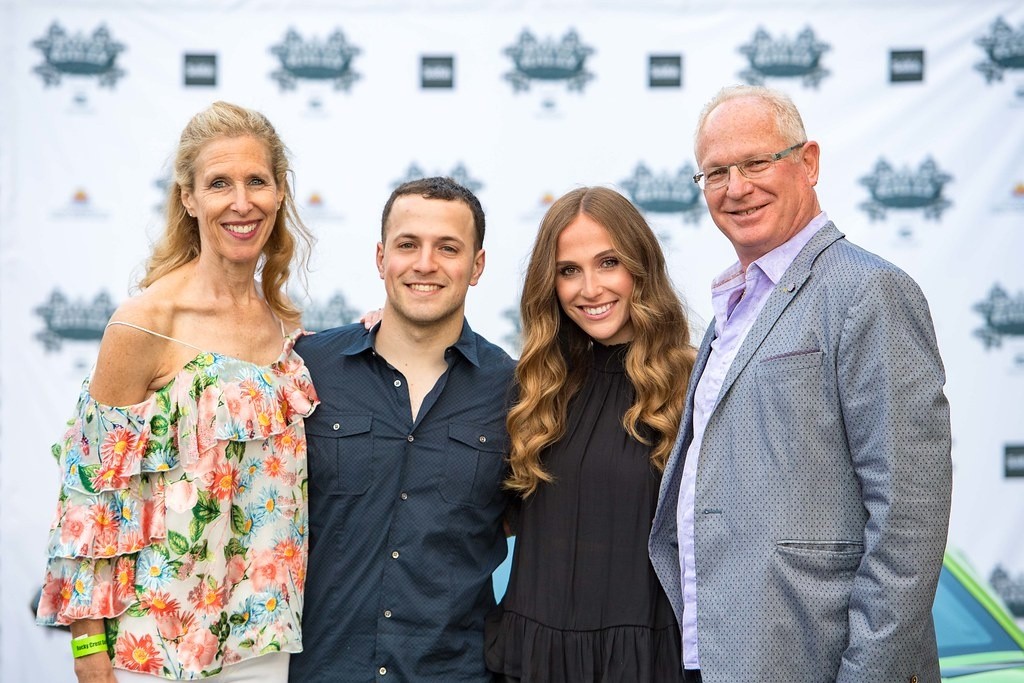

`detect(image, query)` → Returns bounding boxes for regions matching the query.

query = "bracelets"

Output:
[72,634,110,658]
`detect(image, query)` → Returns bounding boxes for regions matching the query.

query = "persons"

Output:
[489,184,697,683]
[644,86,955,683]
[288,175,525,681]
[49,99,386,683]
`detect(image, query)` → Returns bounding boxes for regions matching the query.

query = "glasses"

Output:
[693,142,807,190]
